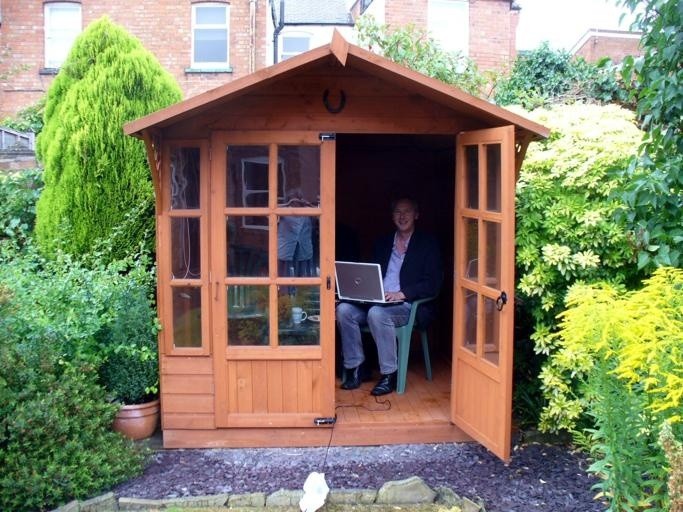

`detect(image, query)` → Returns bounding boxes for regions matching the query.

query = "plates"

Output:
[308,315,321,323]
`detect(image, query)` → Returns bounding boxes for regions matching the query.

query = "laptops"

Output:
[334,261,405,307]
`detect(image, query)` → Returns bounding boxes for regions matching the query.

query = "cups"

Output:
[292,307,308,324]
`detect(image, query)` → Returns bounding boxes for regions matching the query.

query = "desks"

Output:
[278,318,319,345]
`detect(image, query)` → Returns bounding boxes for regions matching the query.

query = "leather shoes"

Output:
[370,371,398,394]
[339,364,367,390]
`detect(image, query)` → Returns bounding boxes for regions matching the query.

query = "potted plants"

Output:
[83,287,163,441]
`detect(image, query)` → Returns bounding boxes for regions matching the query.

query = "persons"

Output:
[277,189,317,294]
[332,195,441,397]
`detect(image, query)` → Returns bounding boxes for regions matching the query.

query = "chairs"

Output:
[226,243,269,346]
[342,270,445,394]
